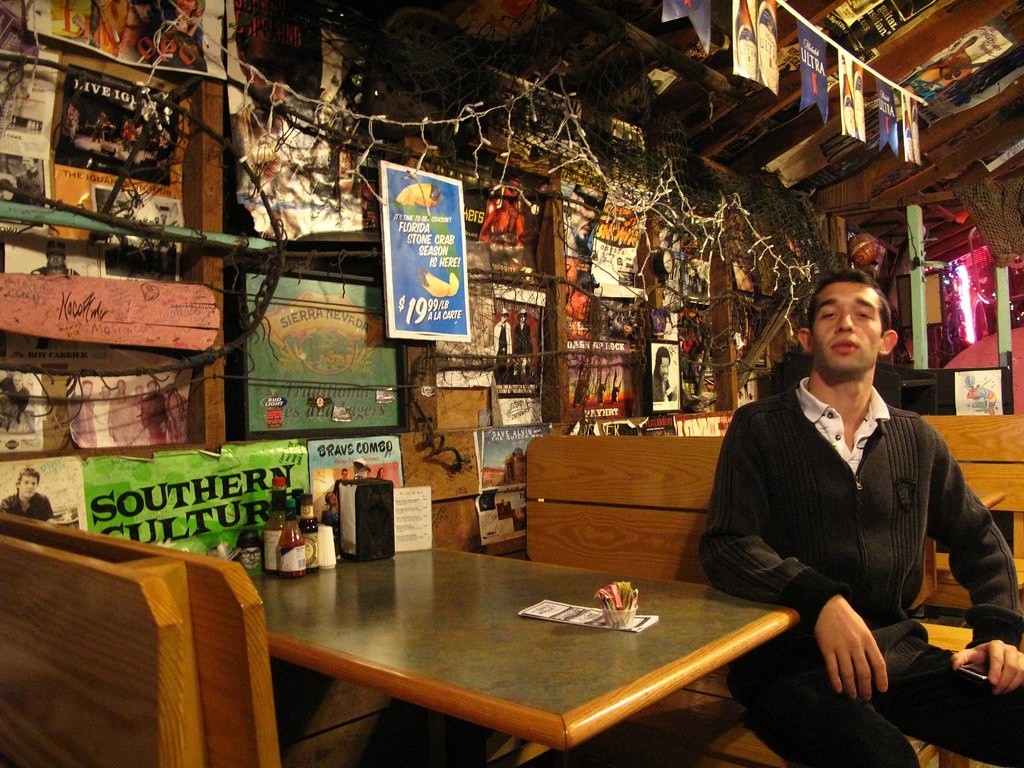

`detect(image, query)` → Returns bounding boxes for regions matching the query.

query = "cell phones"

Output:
[958,662,988,684]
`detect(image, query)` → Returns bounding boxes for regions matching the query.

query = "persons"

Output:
[495,307,533,386]
[921,36,978,90]
[14,156,43,206]
[321,463,386,556]
[652,346,676,401]
[69,379,189,448]
[1,466,56,524]
[0,371,30,430]
[30,241,80,276]
[696,270,1024,768]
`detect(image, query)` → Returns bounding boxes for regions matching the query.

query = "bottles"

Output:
[645,410,734,436]
[237,478,336,577]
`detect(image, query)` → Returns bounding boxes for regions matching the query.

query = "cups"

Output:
[602,606,639,629]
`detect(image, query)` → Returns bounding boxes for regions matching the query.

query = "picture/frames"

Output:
[378,160,471,343]
[224,255,410,442]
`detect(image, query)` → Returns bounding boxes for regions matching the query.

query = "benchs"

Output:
[0,411,1024,768]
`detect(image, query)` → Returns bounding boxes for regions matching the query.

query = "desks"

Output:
[250,550,799,748]
[975,491,1005,510]
